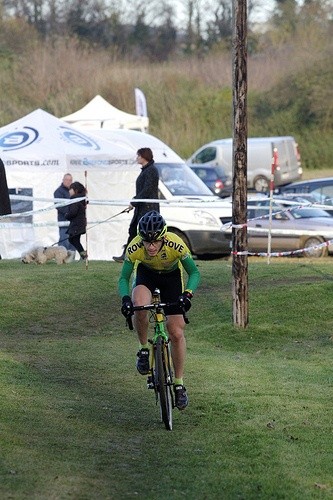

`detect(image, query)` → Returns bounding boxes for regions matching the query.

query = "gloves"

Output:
[121,296,134,318]
[178,291,193,312]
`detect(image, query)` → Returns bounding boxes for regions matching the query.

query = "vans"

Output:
[186,136,303,193]
[82,126,233,258]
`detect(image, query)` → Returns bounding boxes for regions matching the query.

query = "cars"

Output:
[152,162,233,199]
[223,178,333,260]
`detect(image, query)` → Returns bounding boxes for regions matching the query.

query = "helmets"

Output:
[137,211,167,242]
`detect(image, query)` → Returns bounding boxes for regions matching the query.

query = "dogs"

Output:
[22,245,75,266]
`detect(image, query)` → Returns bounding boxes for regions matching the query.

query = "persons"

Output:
[54,174,76,263]
[65,181,89,264]
[118,210,202,410]
[113,147,160,263]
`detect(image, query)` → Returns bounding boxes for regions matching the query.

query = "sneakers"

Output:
[136,348,149,375]
[173,384,187,410]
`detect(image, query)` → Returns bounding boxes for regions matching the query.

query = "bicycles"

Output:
[129,288,185,431]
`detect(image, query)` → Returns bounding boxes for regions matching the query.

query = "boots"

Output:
[113,245,128,263]
[78,251,89,264]
